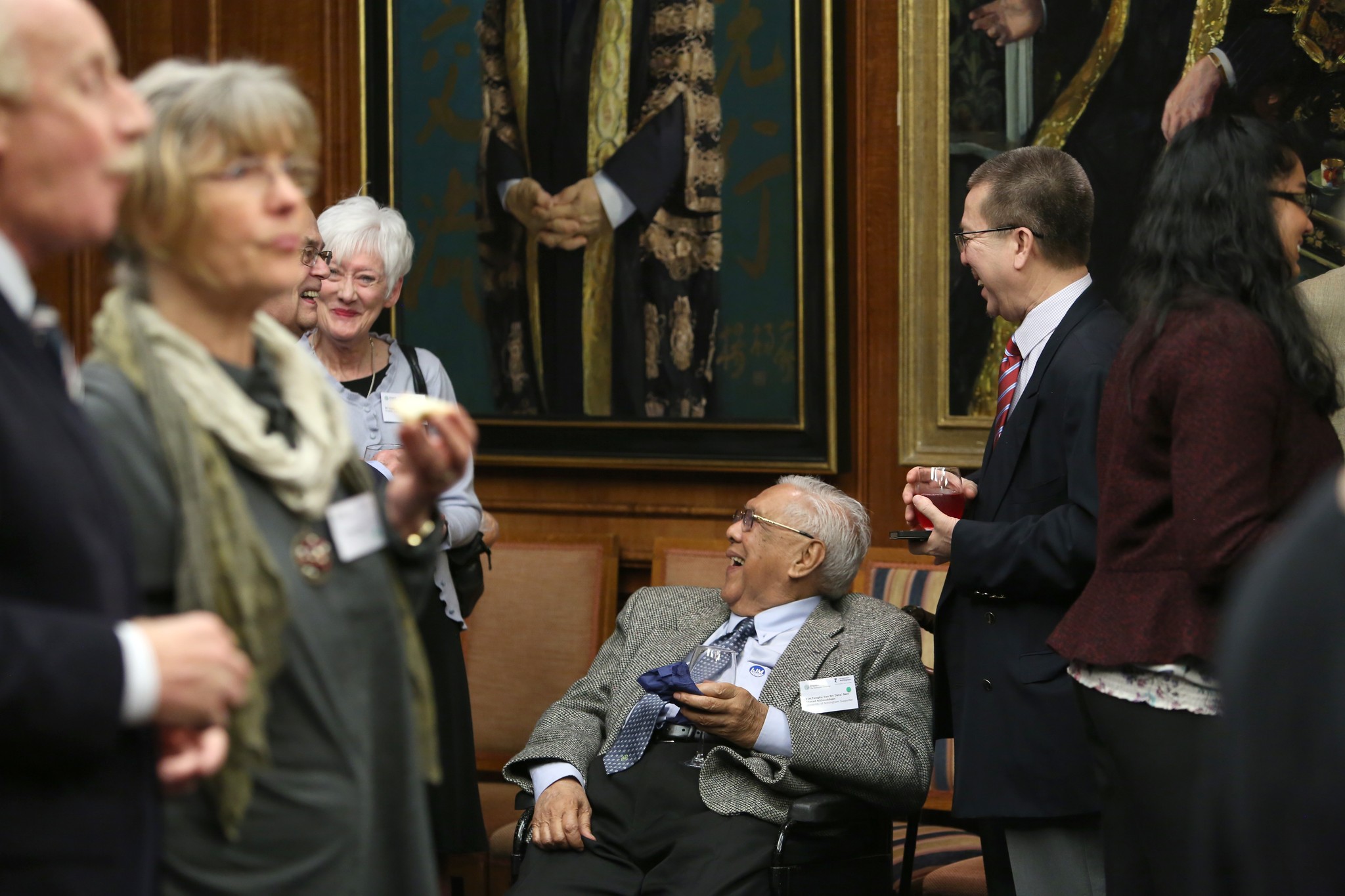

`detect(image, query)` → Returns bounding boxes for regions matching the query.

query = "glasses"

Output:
[730,507,815,539]
[300,246,333,268]
[952,225,1044,254]
[1271,190,1315,217]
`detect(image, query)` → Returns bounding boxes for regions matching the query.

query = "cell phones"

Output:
[888,530,934,538]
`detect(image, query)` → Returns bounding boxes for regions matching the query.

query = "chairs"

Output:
[441,537,619,845]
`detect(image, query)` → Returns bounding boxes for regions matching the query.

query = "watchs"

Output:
[407,520,433,546]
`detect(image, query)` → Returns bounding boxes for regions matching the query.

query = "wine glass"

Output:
[684,645,737,768]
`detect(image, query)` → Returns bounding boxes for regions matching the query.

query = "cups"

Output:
[913,467,964,531]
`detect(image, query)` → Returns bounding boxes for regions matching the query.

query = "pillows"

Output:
[867,562,958,794]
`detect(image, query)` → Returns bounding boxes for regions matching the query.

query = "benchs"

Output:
[648,535,995,895]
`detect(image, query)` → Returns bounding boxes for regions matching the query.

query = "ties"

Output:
[602,615,758,777]
[991,334,1022,449]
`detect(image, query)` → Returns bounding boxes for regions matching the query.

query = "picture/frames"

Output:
[357,1,850,475]
[893,0,1345,471]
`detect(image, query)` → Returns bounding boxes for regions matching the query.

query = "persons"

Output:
[474,0,731,418]
[912,147,1130,896]
[500,476,930,896]
[1049,111,1345,896]
[0,0,255,896]
[966,0,1345,414]
[265,192,506,896]
[87,54,478,896]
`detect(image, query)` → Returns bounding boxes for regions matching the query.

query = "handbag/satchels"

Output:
[396,340,493,617]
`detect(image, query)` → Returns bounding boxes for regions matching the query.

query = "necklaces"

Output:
[313,329,378,399]
